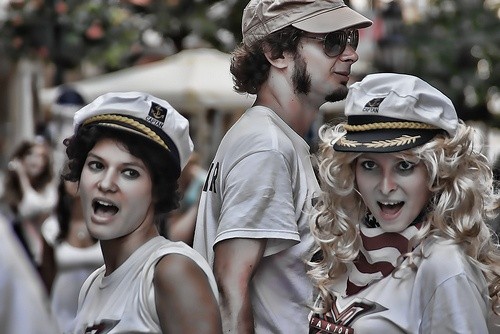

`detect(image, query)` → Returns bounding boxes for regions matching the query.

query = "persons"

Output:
[0,120,207,334]
[308,71,500,334]
[61,92,221,334]
[190,0,373,334]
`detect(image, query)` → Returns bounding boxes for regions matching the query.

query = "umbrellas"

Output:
[38,48,356,171]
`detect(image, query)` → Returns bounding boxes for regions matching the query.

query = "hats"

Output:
[73,92,194,173]
[333,73,458,153]
[242,0,373,48]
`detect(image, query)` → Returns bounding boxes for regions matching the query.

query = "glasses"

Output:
[302,29,359,58]
